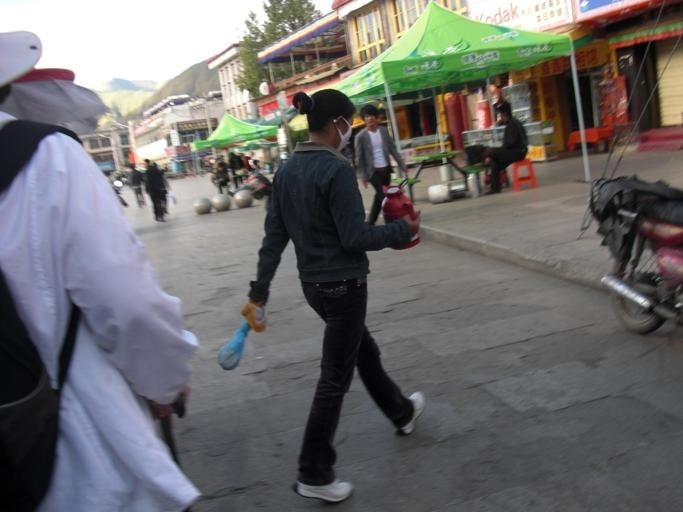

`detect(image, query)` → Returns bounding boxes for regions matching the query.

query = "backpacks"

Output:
[0,118,86,505]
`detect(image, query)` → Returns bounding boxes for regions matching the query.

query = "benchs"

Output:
[452,166,490,195]
[390,177,421,203]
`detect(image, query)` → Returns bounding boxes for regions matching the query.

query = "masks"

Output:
[333,117,354,150]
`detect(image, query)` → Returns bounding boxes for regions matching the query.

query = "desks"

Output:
[405,150,481,203]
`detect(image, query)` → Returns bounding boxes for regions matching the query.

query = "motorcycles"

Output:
[588,176,683,335]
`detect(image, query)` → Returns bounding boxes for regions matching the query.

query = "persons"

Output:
[350,104,410,225]
[477,102,529,196]
[210,150,259,196]
[238,89,428,506]
[140,158,166,222]
[0,26,201,512]
[127,162,145,208]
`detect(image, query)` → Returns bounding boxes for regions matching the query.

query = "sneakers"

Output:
[293,478,353,502]
[394,391,426,435]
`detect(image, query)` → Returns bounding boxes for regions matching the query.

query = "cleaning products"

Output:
[216,321,251,371]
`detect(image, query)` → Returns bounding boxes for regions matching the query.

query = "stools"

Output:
[513,158,536,192]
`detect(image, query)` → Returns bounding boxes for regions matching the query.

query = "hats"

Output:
[2,30,42,88]
